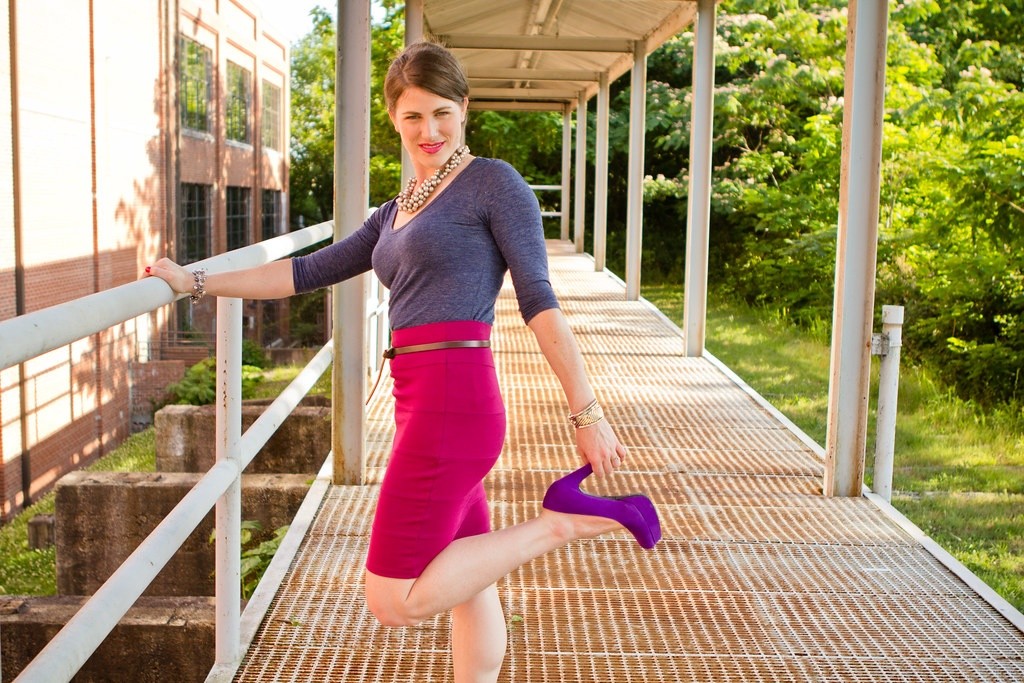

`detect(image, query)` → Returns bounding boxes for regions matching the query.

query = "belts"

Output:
[366,339,491,410]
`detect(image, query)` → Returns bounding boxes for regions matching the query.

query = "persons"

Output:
[141,42,662,683]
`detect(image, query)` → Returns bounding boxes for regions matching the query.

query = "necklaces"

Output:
[396,145,471,213]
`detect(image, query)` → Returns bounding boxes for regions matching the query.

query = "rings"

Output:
[611,457,619,462]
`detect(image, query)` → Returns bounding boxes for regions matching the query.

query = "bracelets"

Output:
[567,398,604,429]
[190,268,207,305]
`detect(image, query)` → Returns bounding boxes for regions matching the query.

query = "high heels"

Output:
[543,462,661,549]
